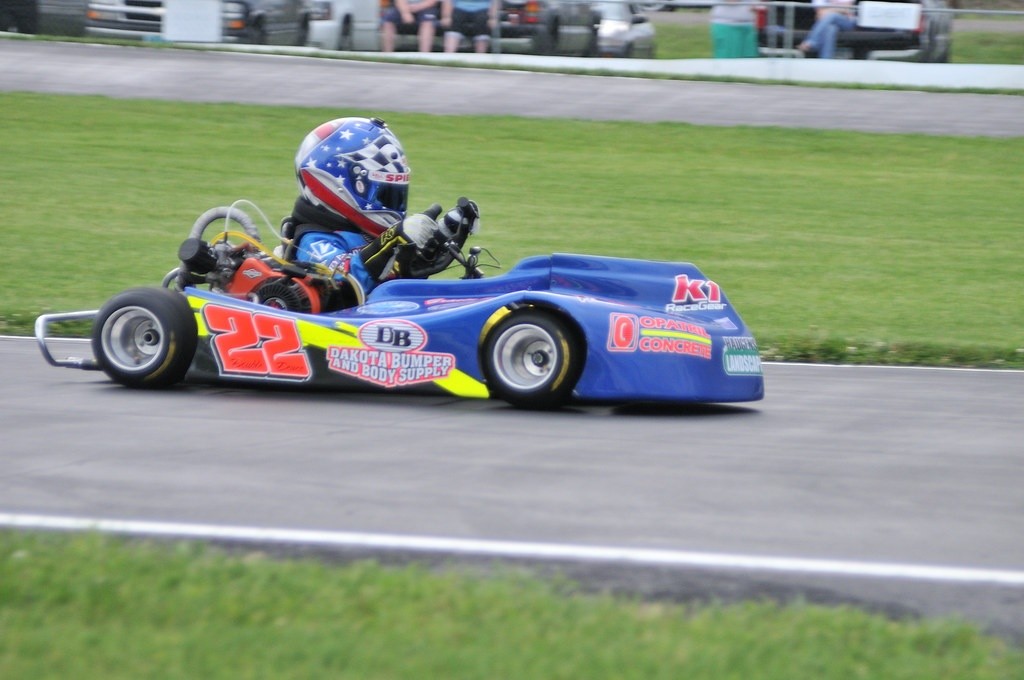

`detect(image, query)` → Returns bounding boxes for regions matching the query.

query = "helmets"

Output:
[295,116,411,238]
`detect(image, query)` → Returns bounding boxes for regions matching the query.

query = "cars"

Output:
[305,0,387,52]
[85,0,308,49]
[399,0,658,60]
[755,0,953,63]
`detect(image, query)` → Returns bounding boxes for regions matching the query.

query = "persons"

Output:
[381,0,438,52]
[441,0,495,53]
[795,0,858,59]
[290,117,480,296]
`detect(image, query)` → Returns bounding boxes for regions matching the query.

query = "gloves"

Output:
[399,213,438,249]
[454,200,480,236]
